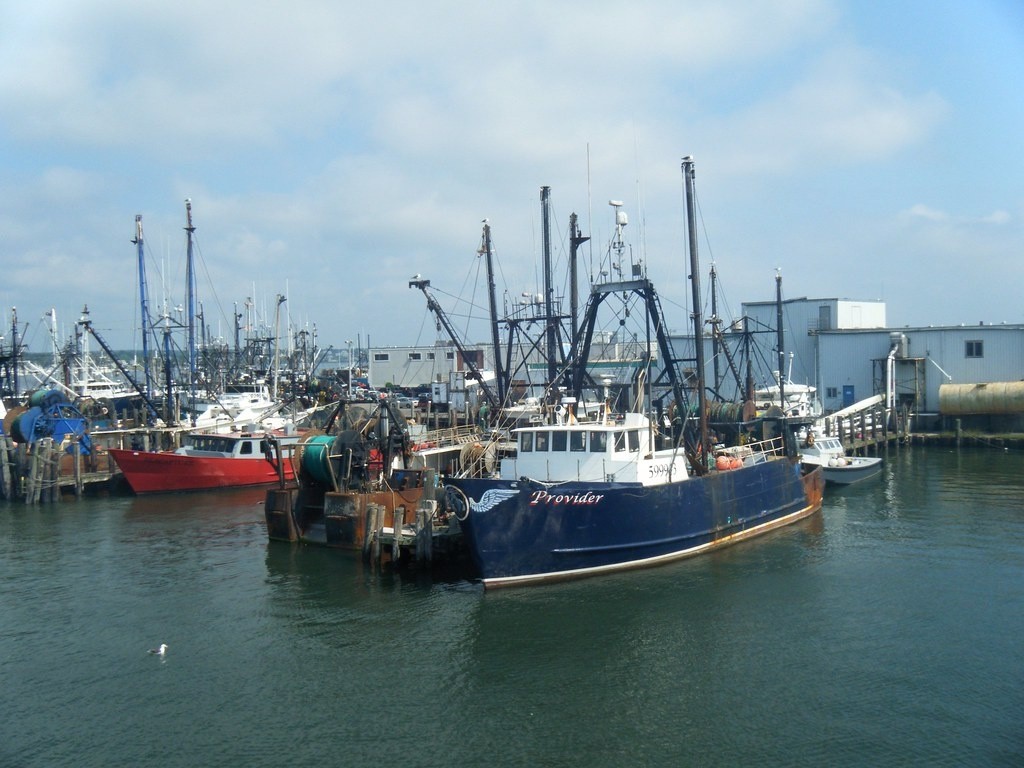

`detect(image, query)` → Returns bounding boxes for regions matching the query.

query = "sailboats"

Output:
[0,152,882,591]
